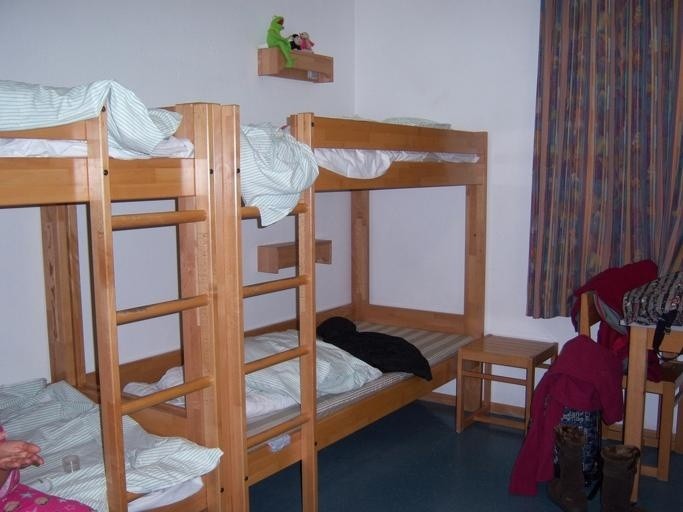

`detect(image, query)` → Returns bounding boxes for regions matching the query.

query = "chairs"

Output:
[577,258,683,483]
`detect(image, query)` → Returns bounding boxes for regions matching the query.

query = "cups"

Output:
[63,455,80,473]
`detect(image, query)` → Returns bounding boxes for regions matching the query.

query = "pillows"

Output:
[383,115,449,129]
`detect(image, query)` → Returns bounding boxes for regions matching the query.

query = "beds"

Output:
[67,102,487,510]
[2,103,226,512]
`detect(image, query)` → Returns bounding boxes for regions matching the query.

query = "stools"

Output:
[457,333,559,437]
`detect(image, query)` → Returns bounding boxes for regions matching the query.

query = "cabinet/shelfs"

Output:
[258,47,334,85]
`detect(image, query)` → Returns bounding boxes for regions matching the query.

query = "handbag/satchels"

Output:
[624,272,683,363]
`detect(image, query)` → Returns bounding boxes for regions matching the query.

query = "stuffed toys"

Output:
[291,33,302,50]
[266,14,294,68]
[301,32,315,53]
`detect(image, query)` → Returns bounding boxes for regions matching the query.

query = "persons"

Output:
[0,425,98,512]
